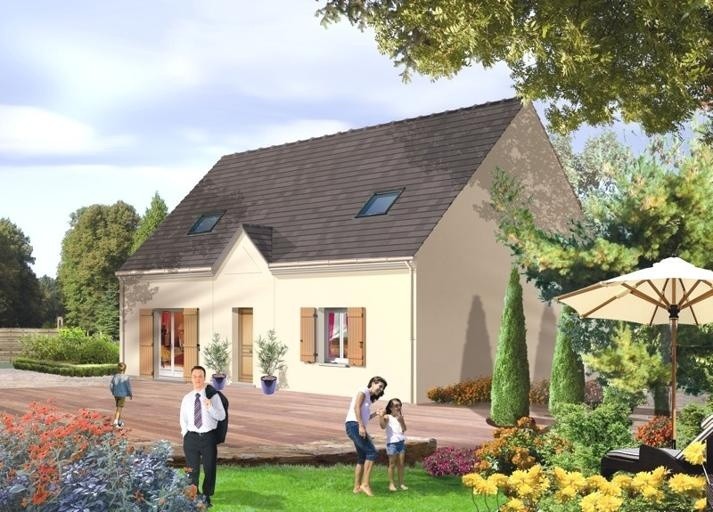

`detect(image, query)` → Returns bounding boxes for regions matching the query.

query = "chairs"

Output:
[598,411,713,497]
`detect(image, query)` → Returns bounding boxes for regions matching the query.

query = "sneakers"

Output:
[114,420,124,427]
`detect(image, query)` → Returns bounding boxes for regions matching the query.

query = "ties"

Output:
[194,393,202,428]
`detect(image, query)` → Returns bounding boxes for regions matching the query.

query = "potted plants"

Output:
[252,328,289,394]
[200,332,233,393]
